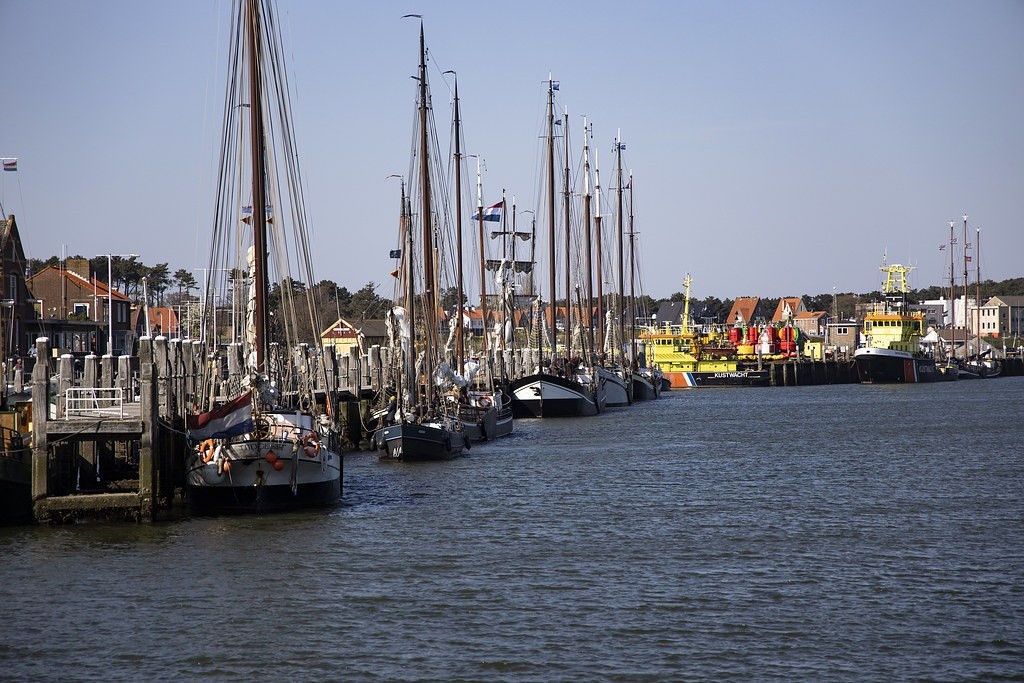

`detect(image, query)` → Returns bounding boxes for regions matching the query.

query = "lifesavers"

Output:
[478,395,491,407]
[200,439,213,462]
[304,433,319,457]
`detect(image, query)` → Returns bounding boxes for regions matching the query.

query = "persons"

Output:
[27,344,37,357]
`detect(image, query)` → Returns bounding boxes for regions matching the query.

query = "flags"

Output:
[186,389,255,444]
[470,200,503,223]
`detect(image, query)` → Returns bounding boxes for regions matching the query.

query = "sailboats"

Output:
[0,14,1023,470]
[182,0,347,520]
